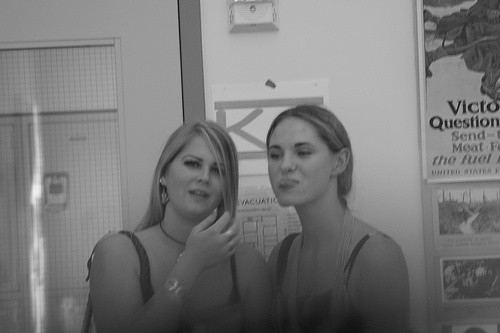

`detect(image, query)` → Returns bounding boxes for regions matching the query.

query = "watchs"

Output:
[165,274,191,303]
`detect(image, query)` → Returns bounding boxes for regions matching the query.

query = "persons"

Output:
[84,119,271,333]
[266,104,411,333]
[444,265,459,285]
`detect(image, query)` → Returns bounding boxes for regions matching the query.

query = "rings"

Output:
[227,241,234,250]
[223,230,234,239]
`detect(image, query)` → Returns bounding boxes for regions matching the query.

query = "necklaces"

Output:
[159,218,187,265]
[285,207,355,333]
